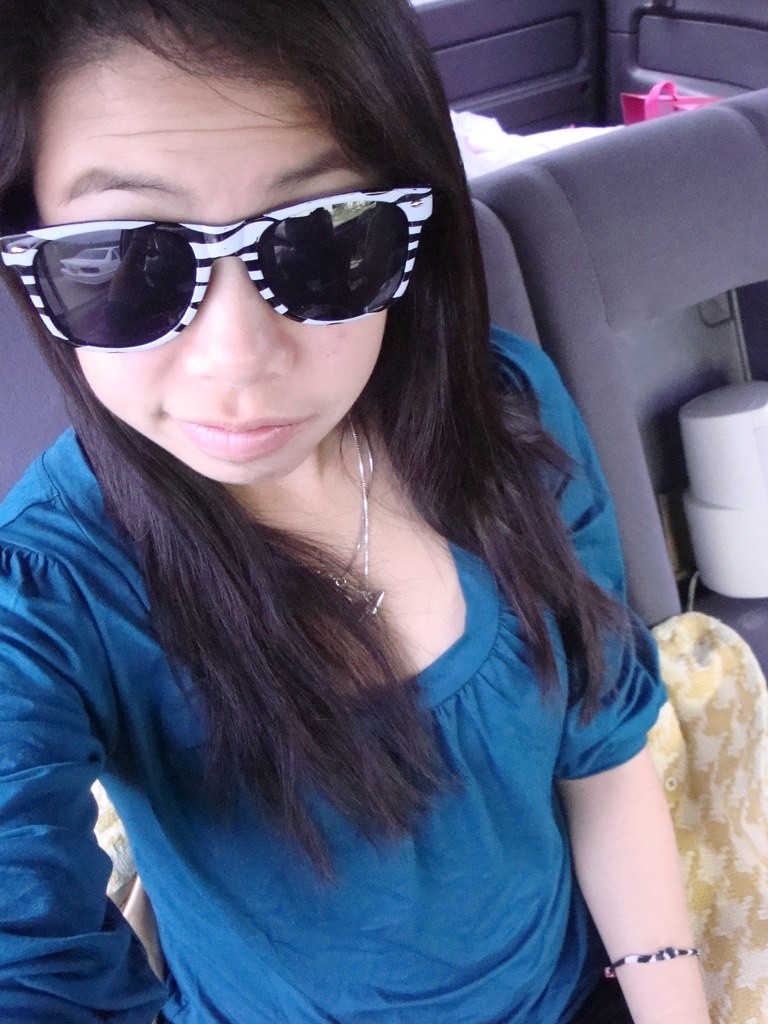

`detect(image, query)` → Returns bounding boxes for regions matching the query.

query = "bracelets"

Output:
[603,947,701,978]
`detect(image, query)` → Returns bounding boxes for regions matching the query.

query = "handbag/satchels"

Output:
[620,81,722,125]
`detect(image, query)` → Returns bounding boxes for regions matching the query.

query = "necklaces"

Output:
[312,412,387,616]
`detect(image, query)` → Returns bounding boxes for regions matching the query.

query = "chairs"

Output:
[0,198,541,503]
[467,87,768,687]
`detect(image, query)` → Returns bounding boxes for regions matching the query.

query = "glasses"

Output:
[0,186,433,354]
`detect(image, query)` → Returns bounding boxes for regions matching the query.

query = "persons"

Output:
[0,2,704,1024]
